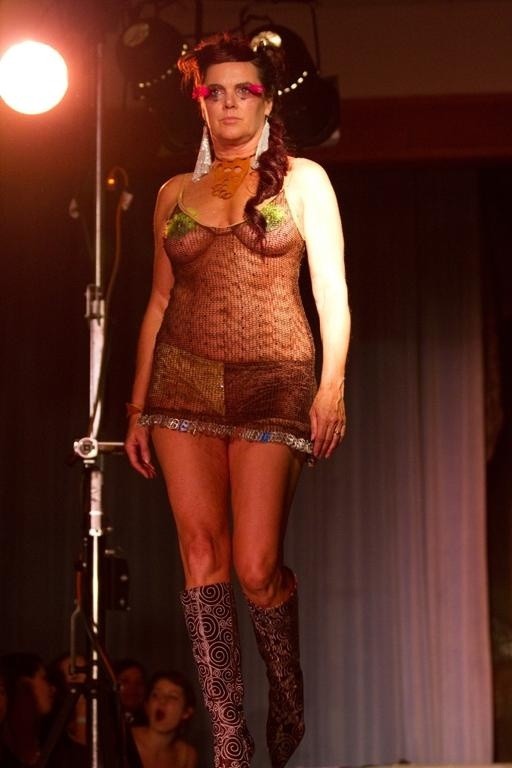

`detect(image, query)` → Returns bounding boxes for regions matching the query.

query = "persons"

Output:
[124,33,352,768]
[0,651,199,768]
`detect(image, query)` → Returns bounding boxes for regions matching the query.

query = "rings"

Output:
[334,430,341,435]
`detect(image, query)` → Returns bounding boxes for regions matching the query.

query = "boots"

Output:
[179,582,257,768]
[239,565,306,768]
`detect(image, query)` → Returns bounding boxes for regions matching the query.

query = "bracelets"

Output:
[125,402,143,418]
